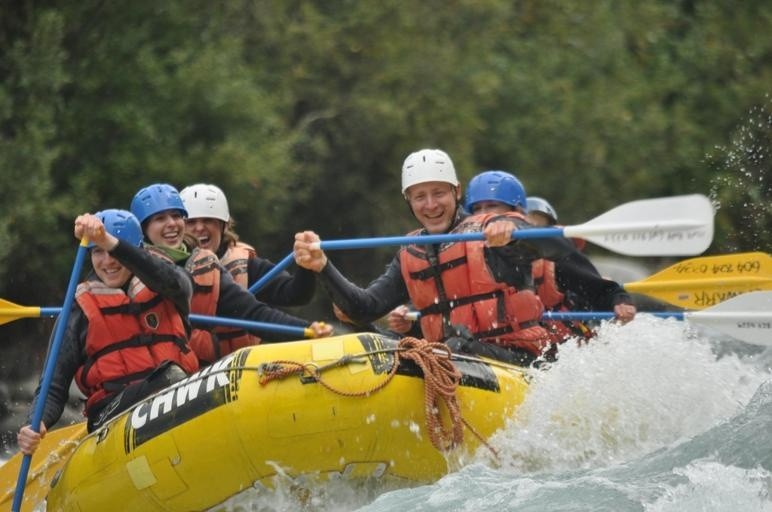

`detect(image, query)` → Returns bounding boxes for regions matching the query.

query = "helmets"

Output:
[402,149,458,195]
[131,182,230,224]
[84,210,143,250]
[466,171,557,221]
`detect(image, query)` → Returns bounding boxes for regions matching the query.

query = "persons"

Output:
[296,148,580,370]
[175,178,322,370]
[130,182,337,372]
[523,196,559,230]
[385,168,635,343]
[16,207,199,461]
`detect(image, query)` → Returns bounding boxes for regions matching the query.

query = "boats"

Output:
[47,333,528,511]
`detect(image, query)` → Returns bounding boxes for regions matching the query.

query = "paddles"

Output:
[1,297,326,340]
[301,193,715,258]
[615,252,772,312]
[402,288,772,347]
[0,252,292,512]
